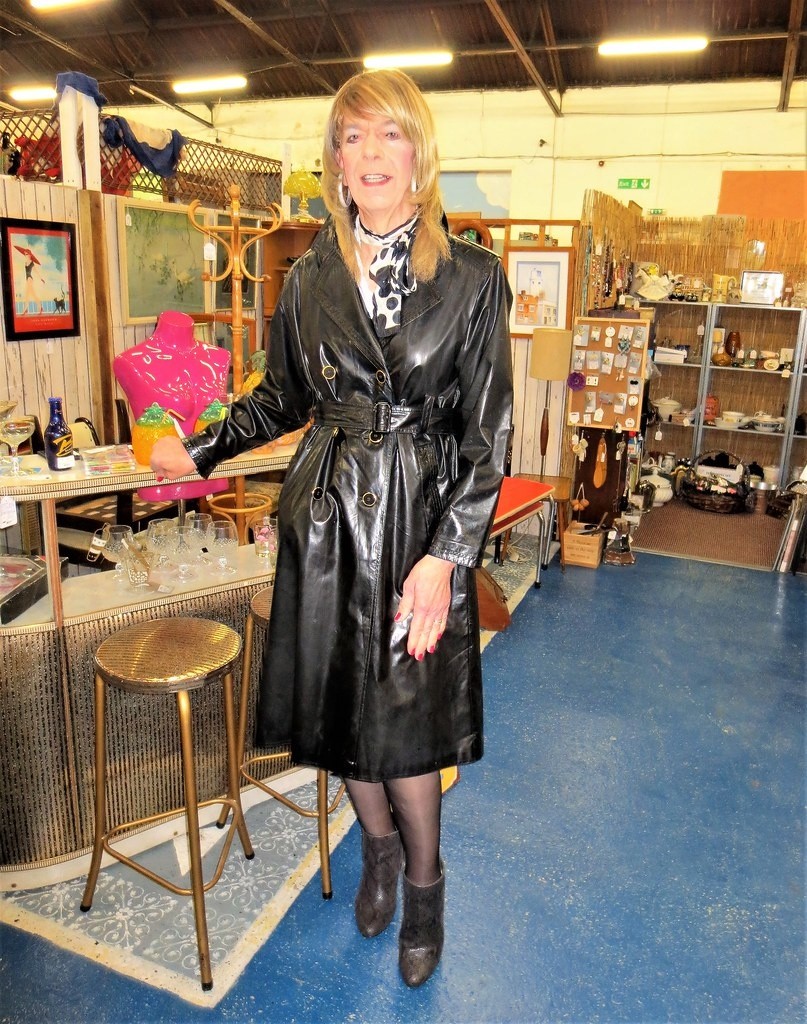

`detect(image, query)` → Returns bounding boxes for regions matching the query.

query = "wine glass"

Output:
[101,512,239,595]
[0,400,19,472]
[0,415,35,478]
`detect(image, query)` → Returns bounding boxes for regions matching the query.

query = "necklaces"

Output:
[581,226,634,317]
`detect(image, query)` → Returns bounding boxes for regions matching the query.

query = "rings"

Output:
[434,620,442,624]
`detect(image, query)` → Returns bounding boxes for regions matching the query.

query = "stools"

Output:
[477,474,572,589]
[217,584,349,900]
[79,616,255,990]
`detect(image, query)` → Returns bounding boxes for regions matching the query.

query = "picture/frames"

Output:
[0,217,81,342]
[116,196,212,326]
[502,245,577,339]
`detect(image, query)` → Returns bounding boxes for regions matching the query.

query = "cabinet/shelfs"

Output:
[259,218,322,352]
[636,296,807,497]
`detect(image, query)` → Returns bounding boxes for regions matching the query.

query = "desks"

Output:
[1,425,314,624]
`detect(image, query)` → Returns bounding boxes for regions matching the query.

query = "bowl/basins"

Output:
[722,411,745,423]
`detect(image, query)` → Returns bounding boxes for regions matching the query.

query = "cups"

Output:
[253,518,279,568]
[710,274,737,304]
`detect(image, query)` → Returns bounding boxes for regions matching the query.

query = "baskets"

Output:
[682,449,751,513]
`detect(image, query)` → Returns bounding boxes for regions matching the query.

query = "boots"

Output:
[400,856,445,989]
[356,824,402,936]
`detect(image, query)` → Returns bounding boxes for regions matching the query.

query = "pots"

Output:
[651,396,683,422]
[751,414,785,433]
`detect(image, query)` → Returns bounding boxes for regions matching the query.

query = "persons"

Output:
[150,70,513,988]
[113,310,231,501]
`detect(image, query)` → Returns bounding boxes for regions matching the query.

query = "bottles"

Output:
[44,396,74,472]
